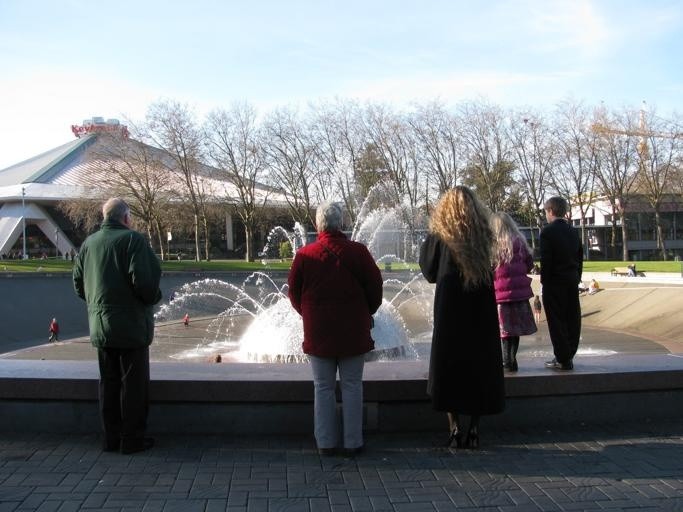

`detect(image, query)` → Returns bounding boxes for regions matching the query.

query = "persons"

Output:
[534,296,541,324]
[489,212,537,374]
[532,264,540,276]
[419,185,506,452]
[49,318,59,343]
[628,264,635,277]
[589,279,599,294]
[183,313,189,327]
[176,248,181,262]
[71,249,75,261]
[288,200,383,457]
[2,251,24,260]
[73,197,162,454]
[539,197,583,370]
[578,280,586,294]
[66,251,68,260]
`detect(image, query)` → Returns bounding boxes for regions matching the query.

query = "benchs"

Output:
[611,266,630,278]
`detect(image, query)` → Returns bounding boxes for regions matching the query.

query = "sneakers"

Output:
[101,434,156,456]
[317,440,368,456]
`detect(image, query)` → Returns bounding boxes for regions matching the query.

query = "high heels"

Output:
[443,424,479,450]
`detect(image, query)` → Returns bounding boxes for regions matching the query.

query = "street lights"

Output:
[22,188,26,259]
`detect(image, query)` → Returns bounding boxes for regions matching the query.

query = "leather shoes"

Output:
[542,357,574,372]
[502,359,519,375]
